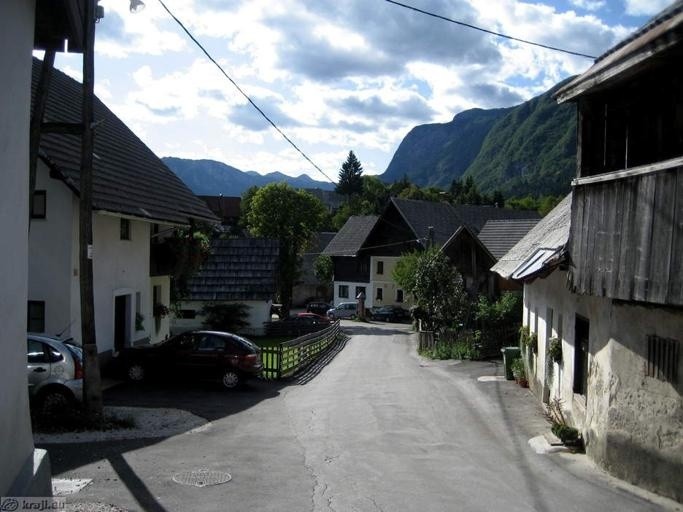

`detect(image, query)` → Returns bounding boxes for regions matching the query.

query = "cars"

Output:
[304,295,411,324]
[25,331,84,422]
[116,328,267,391]
[262,312,329,339]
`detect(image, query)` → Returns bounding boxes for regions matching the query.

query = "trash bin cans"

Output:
[501,347,521,380]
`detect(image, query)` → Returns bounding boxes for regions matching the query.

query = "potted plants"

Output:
[511,357,528,388]
